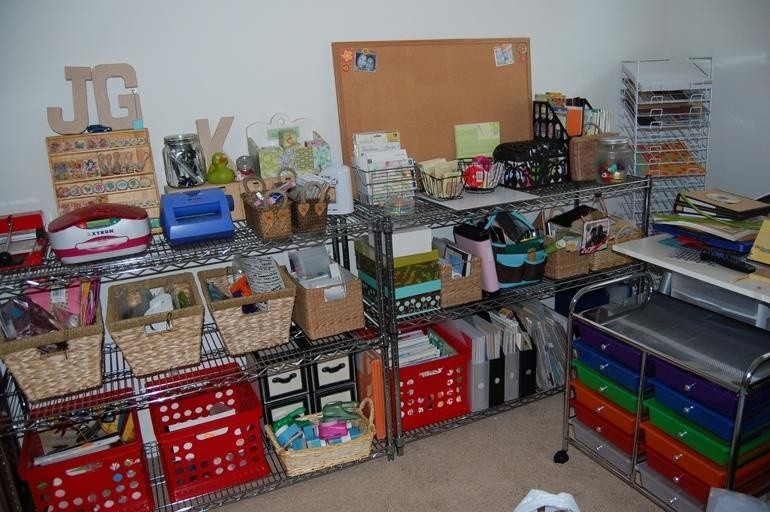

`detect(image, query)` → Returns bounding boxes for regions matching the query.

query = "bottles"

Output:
[595,137,634,187]
[162,133,207,189]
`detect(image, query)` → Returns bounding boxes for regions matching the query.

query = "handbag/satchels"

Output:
[569,120,621,183]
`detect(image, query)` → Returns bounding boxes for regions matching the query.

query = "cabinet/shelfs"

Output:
[3,194,395,509]
[338,174,651,458]
[554,231,769,509]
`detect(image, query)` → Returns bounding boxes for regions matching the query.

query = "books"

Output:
[439,244,471,279]
[533,92,609,141]
[33,431,125,512]
[299,263,347,302]
[376,300,578,418]
[622,60,712,178]
[296,173,330,203]
[352,130,463,206]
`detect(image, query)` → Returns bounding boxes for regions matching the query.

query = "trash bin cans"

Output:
[515,490,580,512]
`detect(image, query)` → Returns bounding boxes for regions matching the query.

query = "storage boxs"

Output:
[357,273,441,320]
[368,223,432,258]
[354,240,441,290]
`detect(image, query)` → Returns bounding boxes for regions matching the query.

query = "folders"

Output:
[673,191,754,220]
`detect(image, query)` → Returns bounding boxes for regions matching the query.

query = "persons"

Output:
[356,53,375,72]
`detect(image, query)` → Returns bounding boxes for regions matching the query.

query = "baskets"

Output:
[105,271,206,378]
[416,155,505,203]
[273,166,331,235]
[280,255,366,342]
[548,210,644,273]
[262,397,377,477]
[432,238,484,309]
[543,229,592,280]
[196,257,297,357]
[0,297,103,404]
[240,174,294,243]
